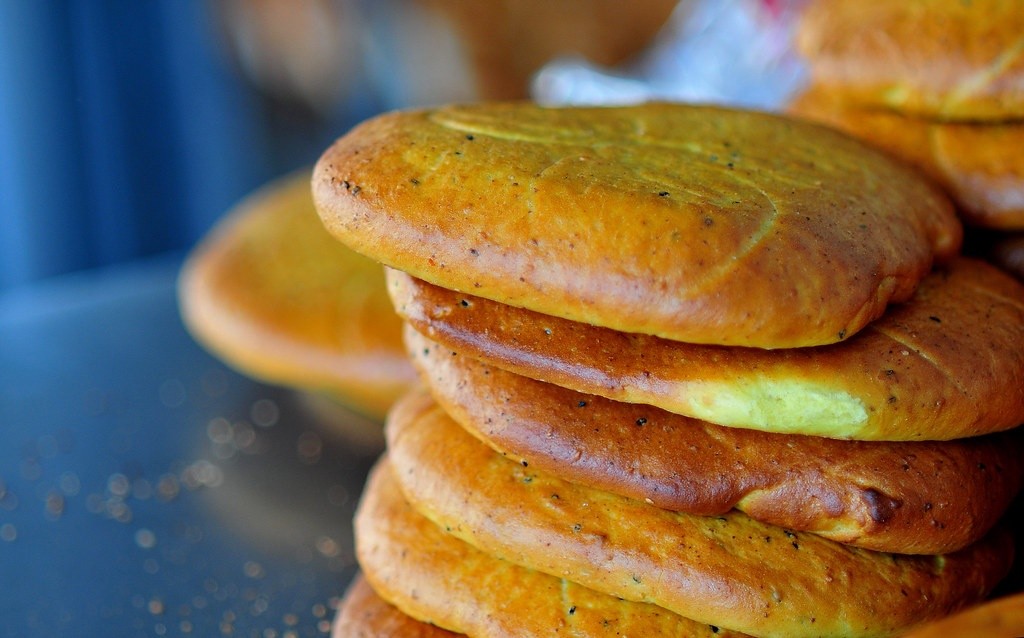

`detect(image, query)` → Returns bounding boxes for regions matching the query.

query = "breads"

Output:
[177,0,1024,638]
